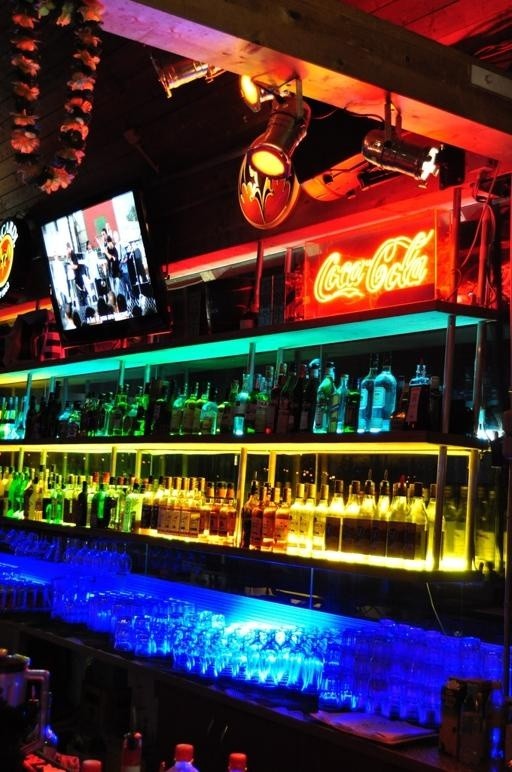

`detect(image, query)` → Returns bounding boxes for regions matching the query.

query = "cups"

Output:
[322,618,512,759]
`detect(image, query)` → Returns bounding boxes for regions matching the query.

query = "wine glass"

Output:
[0,527,135,575]
[51,586,328,694]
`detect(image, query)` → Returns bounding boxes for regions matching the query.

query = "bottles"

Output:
[1,347,507,445]
[137,542,231,589]
[81,730,249,772]
[243,468,502,573]
[0,461,239,542]
[0,584,54,611]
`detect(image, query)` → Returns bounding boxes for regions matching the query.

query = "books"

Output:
[309,710,438,746]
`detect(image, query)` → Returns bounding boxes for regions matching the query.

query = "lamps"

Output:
[155,58,441,181]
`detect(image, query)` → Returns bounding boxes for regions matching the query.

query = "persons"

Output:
[64,223,148,332]
[431,357,474,435]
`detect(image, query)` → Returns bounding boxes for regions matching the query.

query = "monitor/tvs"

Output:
[38,187,170,344]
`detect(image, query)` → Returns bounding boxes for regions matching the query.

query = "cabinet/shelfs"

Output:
[0,184,506,584]
